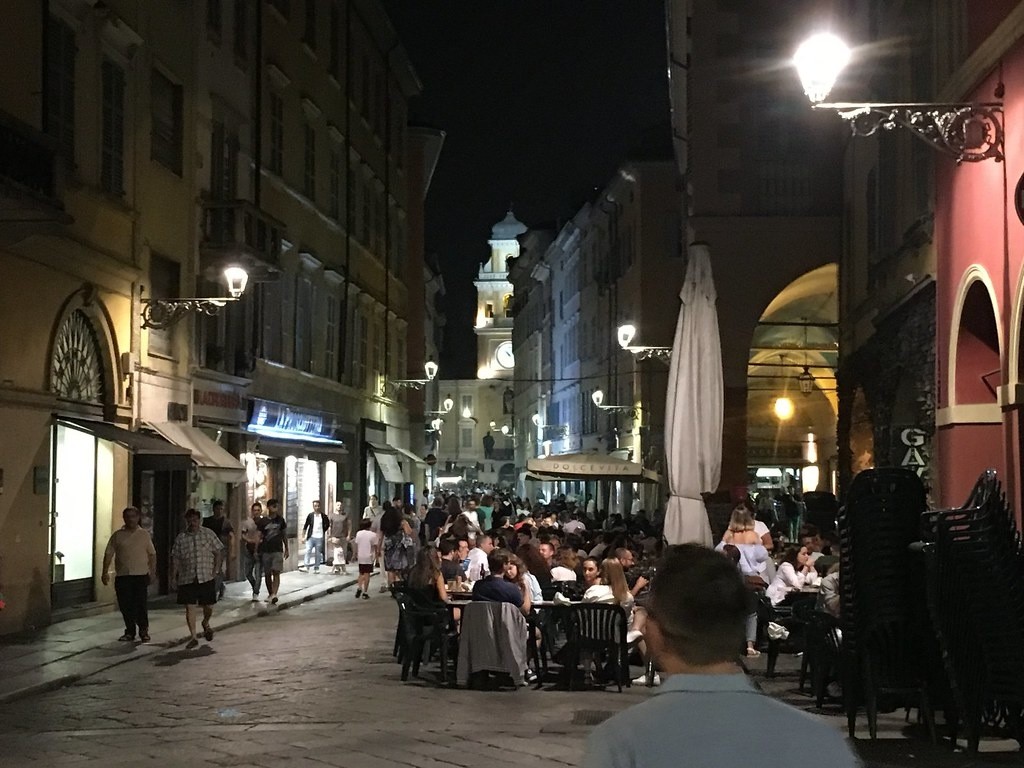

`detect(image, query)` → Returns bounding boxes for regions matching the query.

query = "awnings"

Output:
[519,453,659,481]
[54,416,191,470]
[397,448,425,466]
[149,420,248,483]
[374,452,406,484]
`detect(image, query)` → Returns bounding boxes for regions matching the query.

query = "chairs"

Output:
[380,578,658,695]
[752,467,1024,755]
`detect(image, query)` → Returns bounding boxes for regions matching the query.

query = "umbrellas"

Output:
[661,242,724,549]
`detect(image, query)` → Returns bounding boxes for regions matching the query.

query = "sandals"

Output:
[746,647,761,658]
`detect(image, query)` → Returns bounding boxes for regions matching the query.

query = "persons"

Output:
[353,480,664,688]
[327,501,352,575]
[101,507,156,642]
[202,501,234,600]
[715,473,842,698]
[581,544,856,768]
[299,500,329,574]
[169,509,224,648]
[241,498,289,603]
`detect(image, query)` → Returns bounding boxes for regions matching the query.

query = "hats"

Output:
[468,500,475,505]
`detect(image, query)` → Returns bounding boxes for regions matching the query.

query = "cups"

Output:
[447,579,456,590]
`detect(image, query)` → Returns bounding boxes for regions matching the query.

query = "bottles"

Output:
[479,563,486,580]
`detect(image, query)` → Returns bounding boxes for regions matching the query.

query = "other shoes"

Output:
[632,673,661,686]
[139,633,150,641]
[355,587,362,598]
[217,584,226,600]
[264,594,278,604]
[202,621,214,641]
[313,569,319,574]
[362,592,369,599]
[299,566,309,573]
[186,640,198,648]
[119,633,134,640]
[252,592,258,601]
[584,670,614,688]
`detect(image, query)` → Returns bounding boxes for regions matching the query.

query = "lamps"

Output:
[429,398,453,415]
[617,325,672,365]
[490,422,501,432]
[500,426,516,437]
[532,413,568,437]
[793,35,1008,174]
[796,316,815,397]
[774,354,794,420]
[378,362,439,399]
[424,418,445,434]
[590,390,634,416]
[138,266,248,331]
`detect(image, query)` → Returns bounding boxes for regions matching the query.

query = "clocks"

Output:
[495,340,515,369]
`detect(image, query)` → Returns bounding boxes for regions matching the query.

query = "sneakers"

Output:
[524,668,537,681]
